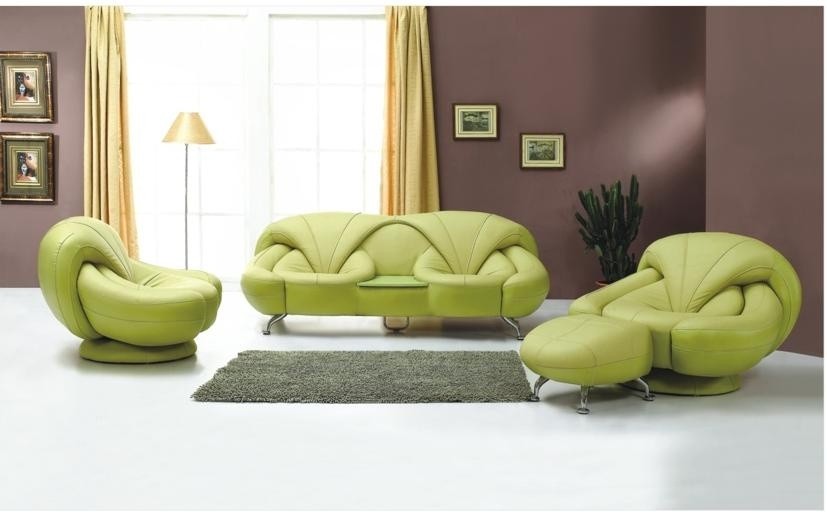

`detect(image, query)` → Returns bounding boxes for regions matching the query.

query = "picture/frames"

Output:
[1,51,54,124]
[451,103,499,141]
[519,133,566,170]
[0,132,55,203]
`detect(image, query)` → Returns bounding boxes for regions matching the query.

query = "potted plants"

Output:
[573,175,643,288]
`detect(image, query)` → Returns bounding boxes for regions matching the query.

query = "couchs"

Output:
[240,212,550,340]
[37,216,220,364]
[520,232,801,414]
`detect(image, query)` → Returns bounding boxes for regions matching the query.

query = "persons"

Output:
[477,114,488,130]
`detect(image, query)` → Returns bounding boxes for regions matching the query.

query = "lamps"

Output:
[163,112,215,272]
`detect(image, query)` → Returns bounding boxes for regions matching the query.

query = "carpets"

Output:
[190,349,533,403]
